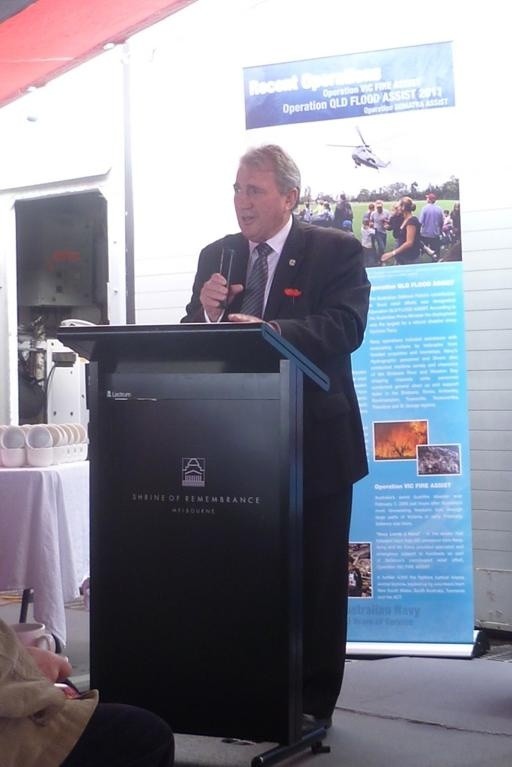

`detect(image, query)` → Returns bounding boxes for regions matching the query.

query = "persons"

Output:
[179,145,371,738]
[0,619,175,767]
[361,194,461,267]
[299,194,355,236]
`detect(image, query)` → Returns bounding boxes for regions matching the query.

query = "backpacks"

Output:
[344,208,353,219]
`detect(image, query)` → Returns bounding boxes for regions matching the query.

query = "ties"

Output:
[239,242,274,319]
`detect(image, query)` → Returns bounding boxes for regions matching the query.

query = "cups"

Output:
[10,622,56,653]
[0,421,89,467]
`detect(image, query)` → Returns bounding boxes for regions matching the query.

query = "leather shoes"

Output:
[302,713,332,735]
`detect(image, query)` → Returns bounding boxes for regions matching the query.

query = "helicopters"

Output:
[325,123,391,170]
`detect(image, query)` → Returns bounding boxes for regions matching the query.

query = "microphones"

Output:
[217,234,240,309]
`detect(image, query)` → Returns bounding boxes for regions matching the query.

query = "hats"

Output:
[376,200,383,207]
[425,194,436,200]
[343,220,351,227]
